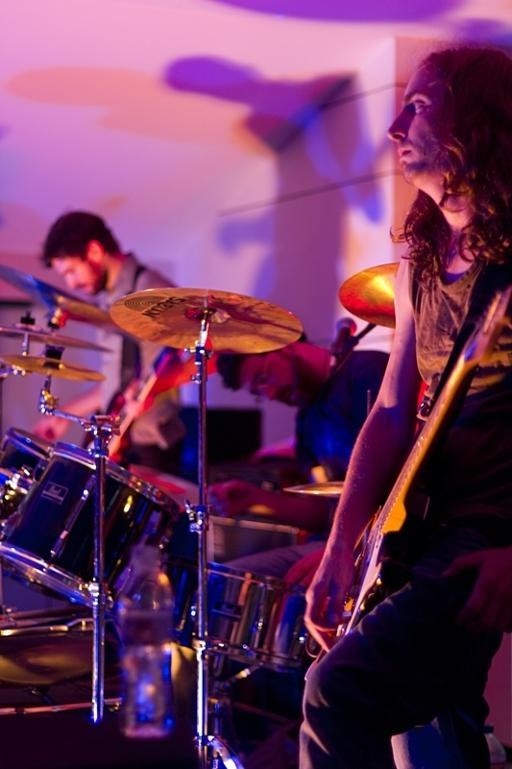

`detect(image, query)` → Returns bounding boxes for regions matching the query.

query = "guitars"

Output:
[319,244,511,654]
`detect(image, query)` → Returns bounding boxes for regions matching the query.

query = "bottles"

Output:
[112,545,180,739]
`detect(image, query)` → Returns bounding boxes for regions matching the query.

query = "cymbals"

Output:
[286,479,341,496]
[0,323,113,357]
[0,349,105,384]
[339,261,400,328]
[106,288,304,357]
[0,298,41,312]
[0,263,114,330]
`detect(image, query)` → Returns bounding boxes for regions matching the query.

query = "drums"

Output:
[204,512,305,578]
[175,554,311,681]
[0,426,53,532]
[3,438,185,618]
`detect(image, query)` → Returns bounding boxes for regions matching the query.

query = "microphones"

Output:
[328,317,357,371]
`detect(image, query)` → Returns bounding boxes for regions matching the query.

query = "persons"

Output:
[30,208,189,483]
[294,48,511,766]
[197,319,390,744]
